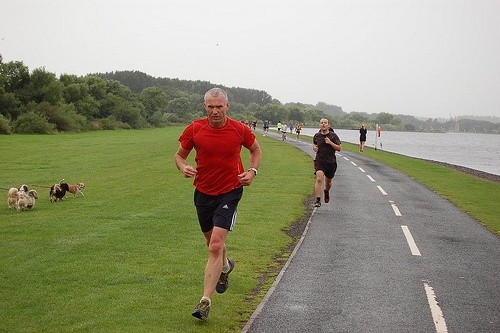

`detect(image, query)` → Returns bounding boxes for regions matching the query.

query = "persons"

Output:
[276,121,281,135]
[295,123,302,140]
[359,124,367,153]
[176,88,262,320]
[290,124,294,134]
[312,116,342,208]
[240,118,270,134]
[281,122,288,142]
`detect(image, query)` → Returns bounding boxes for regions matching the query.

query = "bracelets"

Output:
[248,168,257,176]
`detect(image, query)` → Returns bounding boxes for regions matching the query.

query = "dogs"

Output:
[7,184,29,210]
[59,178,86,198]
[16,189,39,212]
[49,183,70,204]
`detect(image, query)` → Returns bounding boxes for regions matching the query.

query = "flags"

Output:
[377,126,382,148]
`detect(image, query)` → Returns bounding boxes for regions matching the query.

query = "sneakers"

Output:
[216,257,234,293]
[324,190,329,203]
[191,299,210,320]
[315,196,321,207]
